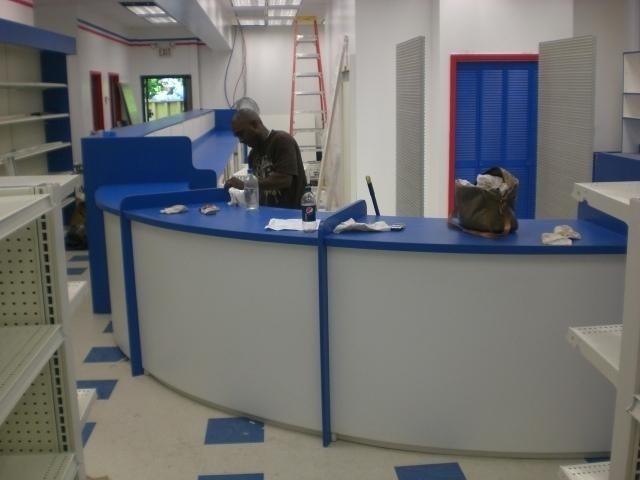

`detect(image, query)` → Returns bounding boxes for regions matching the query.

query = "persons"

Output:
[222,109,309,209]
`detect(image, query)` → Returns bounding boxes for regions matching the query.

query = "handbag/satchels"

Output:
[448,166,521,241]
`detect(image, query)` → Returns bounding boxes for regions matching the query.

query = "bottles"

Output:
[301,185,318,233]
[243,169,260,211]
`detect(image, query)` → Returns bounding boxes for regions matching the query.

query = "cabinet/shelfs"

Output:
[620,47,640,161]
[0,173,93,476]
[555,176,640,480]
[0,18,78,229]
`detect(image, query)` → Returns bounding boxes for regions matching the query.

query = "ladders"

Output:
[290,16,328,191]
